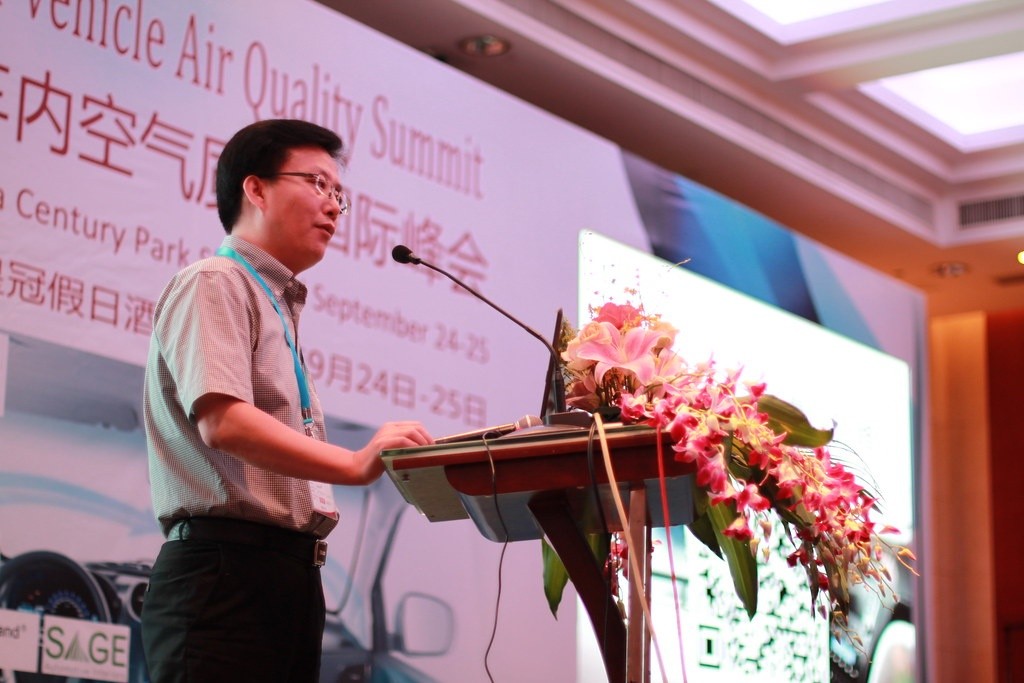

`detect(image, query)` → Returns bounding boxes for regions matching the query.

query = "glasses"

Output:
[276,172,351,216]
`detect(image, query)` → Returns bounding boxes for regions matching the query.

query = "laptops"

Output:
[540,309,563,420]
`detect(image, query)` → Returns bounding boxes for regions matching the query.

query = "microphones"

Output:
[392,245,592,429]
[434,416,542,444]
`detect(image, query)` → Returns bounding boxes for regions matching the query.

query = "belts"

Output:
[167,519,328,566]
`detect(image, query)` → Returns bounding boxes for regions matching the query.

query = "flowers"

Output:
[542,263,920,662]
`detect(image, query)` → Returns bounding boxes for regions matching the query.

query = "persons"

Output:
[140,120,435,683]
